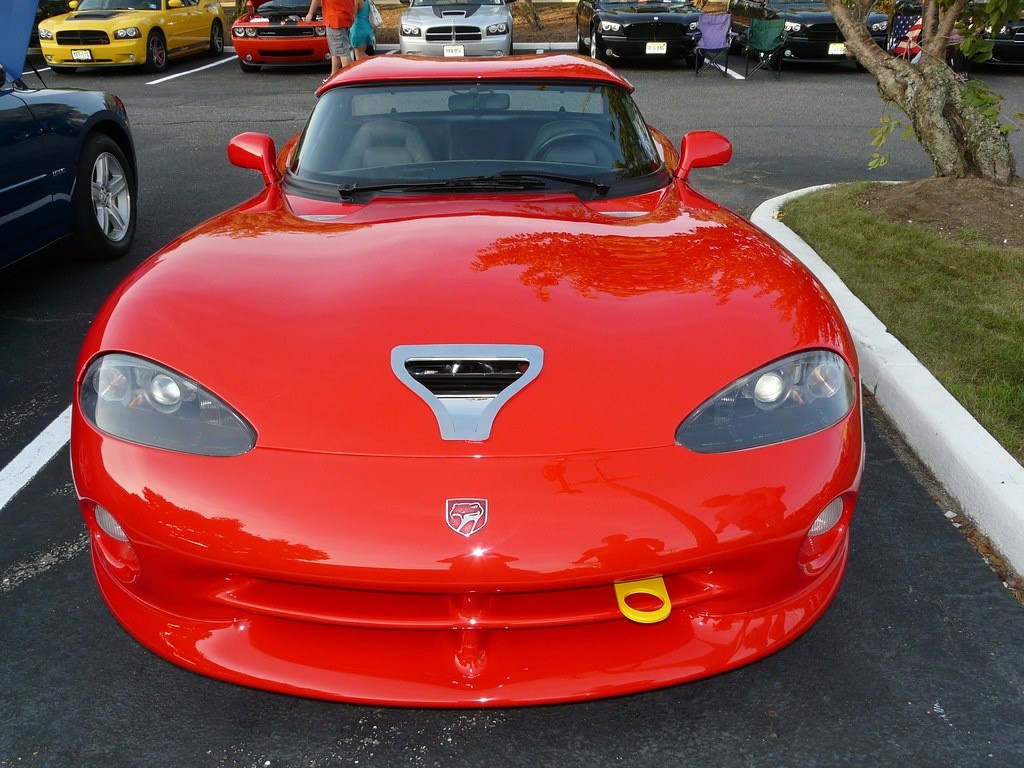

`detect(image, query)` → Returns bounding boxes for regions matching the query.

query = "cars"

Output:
[399,1,514,55]
[940,1,1023,73]
[1,2,139,264]
[38,1,225,70]
[230,1,376,72]
[576,0,706,67]
[725,0,891,70]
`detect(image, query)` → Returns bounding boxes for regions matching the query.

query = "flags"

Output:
[889,13,922,54]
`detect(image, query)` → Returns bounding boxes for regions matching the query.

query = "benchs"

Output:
[341,114,610,160]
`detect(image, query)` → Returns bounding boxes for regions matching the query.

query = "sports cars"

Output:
[71,55,865,710]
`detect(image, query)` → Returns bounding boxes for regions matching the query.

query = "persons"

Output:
[305,0,375,85]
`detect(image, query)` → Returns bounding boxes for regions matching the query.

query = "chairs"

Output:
[523,119,619,166]
[334,120,436,172]
[734,17,789,82]
[686,14,739,78]
[883,13,922,61]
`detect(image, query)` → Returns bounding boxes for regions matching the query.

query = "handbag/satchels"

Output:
[369,5,383,27]
